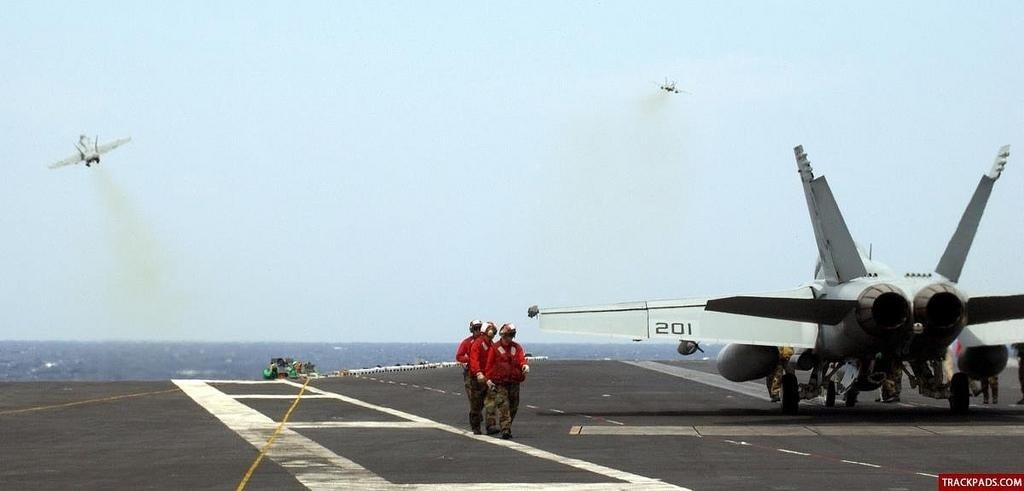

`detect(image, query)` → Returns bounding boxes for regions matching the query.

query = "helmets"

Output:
[470,320,482,332]
[500,324,516,335]
[480,321,497,337]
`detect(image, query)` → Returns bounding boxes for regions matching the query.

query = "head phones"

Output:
[487,322,493,337]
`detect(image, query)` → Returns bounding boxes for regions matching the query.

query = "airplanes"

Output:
[651,77,686,95]
[44,134,134,173]
[523,138,1023,416]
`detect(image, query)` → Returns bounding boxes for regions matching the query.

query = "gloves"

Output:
[522,364,529,375]
[487,380,496,391]
[476,372,487,384]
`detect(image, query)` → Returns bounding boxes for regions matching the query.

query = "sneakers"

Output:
[876,397,899,403]
[1017,398,1024,404]
[984,398,988,403]
[771,398,780,402]
[472,413,512,439]
[993,398,997,403]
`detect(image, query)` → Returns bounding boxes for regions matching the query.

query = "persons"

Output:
[469,321,500,435]
[458,322,484,423]
[764,348,793,404]
[484,324,531,441]
[881,365,902,404]
[1012,344,1023,405]
[981,375,1001,404]
